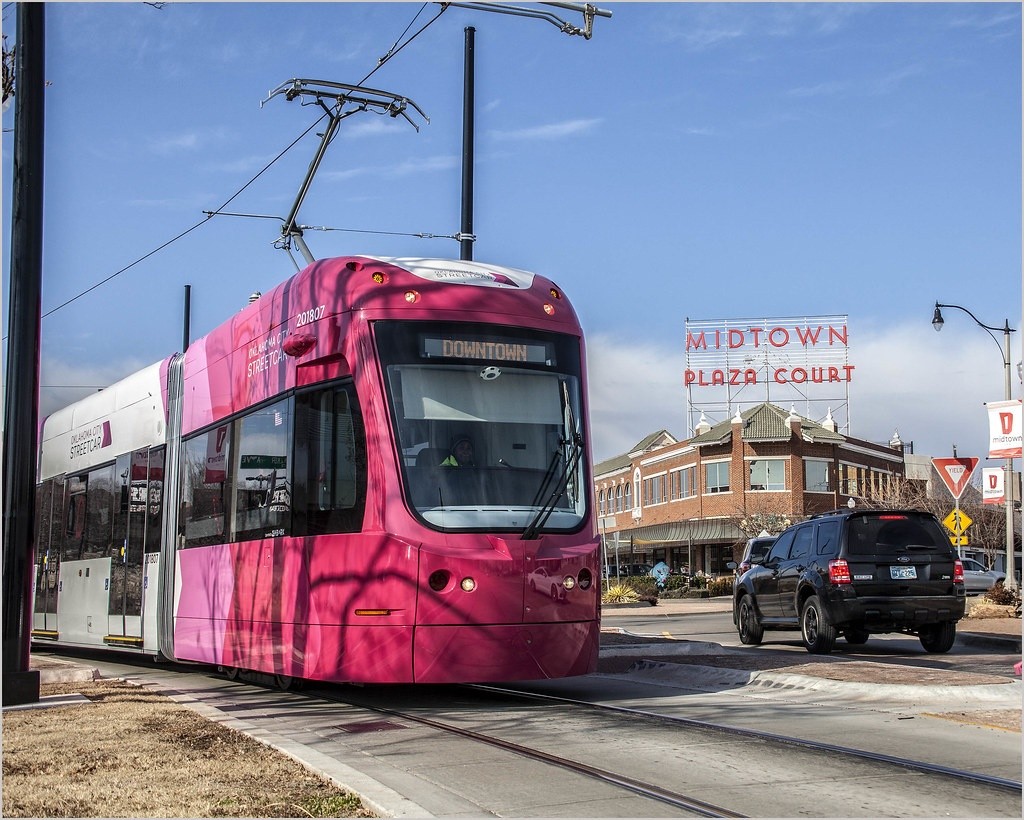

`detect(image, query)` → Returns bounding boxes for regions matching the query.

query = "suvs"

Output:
[730,534,781,627]
[732,505,967,655]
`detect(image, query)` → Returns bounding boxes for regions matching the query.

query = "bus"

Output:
[29,79,605,688]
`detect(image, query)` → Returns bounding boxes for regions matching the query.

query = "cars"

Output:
[957,558,1009,596]
[604,564,657,580]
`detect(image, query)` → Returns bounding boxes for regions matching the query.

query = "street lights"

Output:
[932,301,1016,596]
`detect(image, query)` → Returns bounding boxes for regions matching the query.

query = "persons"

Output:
[438,434,479,466]
[695,569,711,578]
[683,564,689,572]
